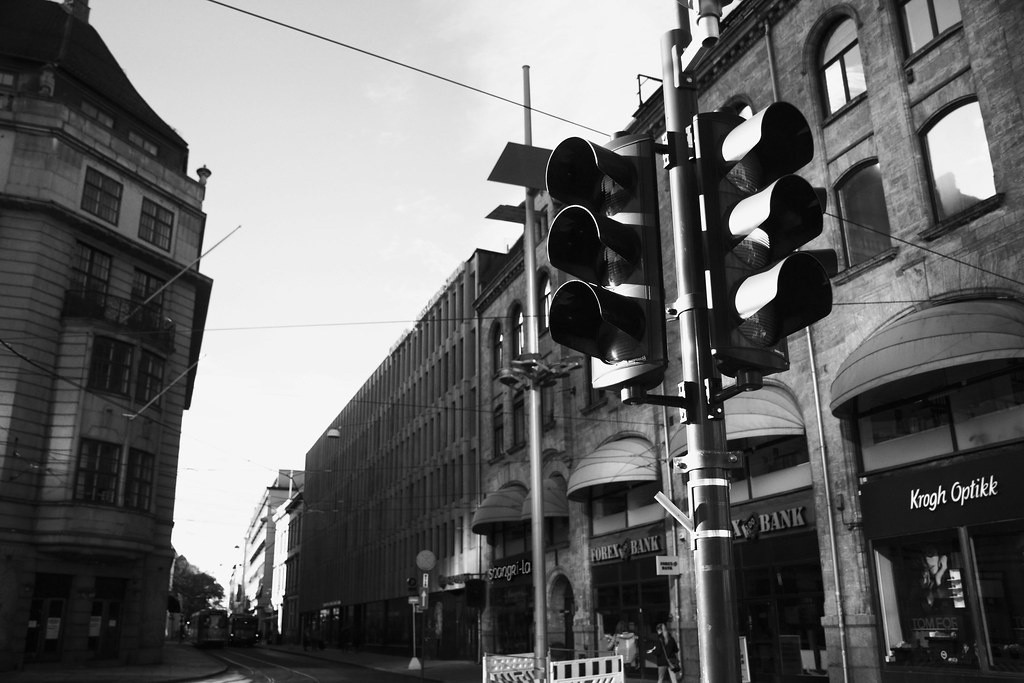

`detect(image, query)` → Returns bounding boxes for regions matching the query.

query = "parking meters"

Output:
[405,577,423,671]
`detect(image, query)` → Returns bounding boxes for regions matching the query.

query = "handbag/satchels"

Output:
[667,658,682,673]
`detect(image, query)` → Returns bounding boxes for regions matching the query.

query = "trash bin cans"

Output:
[923,634,959,666]
[890,645,929,664]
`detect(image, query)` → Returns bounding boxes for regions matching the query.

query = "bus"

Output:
[226,613,259,649]
[191,609,230,649]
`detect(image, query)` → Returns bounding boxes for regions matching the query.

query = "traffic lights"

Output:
[693,101,838,379]
[548,136,670,400]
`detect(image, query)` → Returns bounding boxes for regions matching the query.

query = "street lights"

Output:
[498,350,580,683]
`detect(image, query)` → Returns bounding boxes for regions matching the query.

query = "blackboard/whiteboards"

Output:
[780,635,805,677]
[739,636,751,682]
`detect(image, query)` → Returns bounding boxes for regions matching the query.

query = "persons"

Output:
[613,621,638,668]
[918,543,948,608]
[652,623,681,683]
